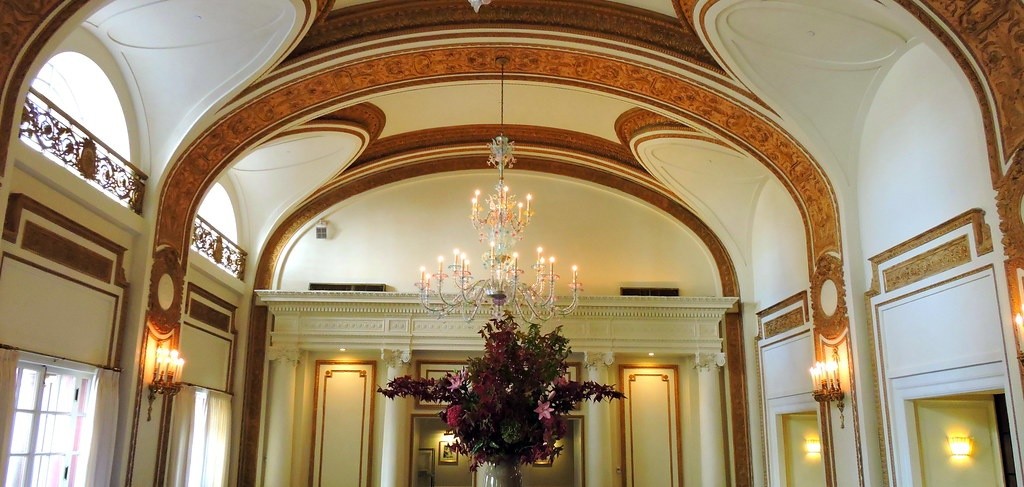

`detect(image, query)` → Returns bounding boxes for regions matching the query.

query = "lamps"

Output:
[809,360,844,401]
[149,348,184,395]
[414,56,583,327]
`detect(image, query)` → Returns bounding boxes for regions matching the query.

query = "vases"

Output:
[475,461,522,487]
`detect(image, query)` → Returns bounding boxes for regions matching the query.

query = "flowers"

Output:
[377,311,627,465]
